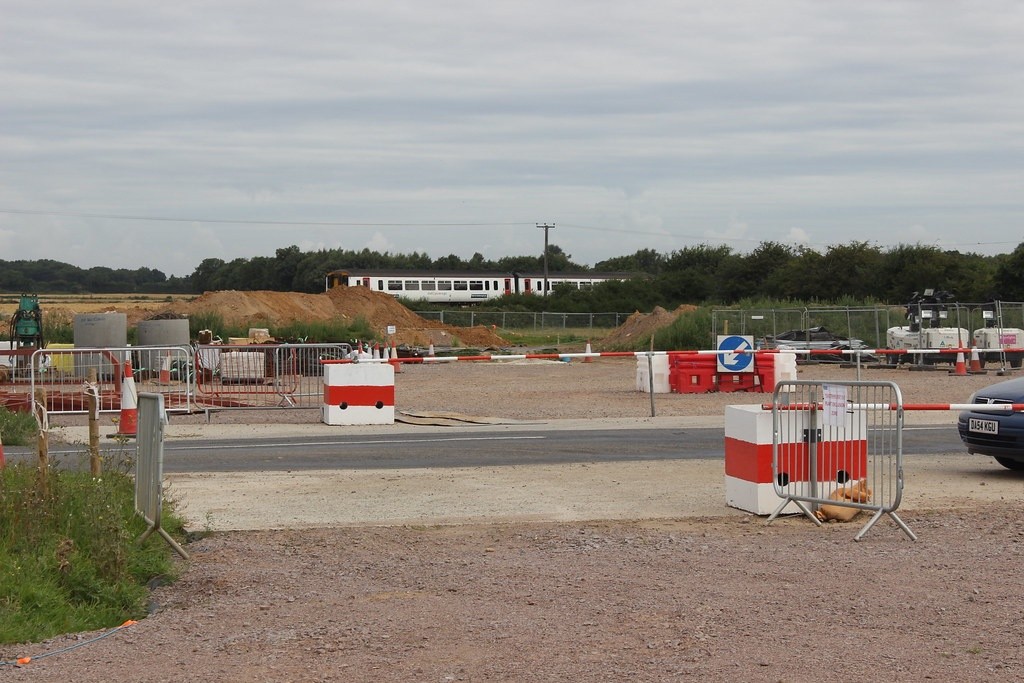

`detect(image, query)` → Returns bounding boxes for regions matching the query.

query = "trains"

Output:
[326,270,635,304]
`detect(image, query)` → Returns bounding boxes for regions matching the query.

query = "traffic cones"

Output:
[423,339,439,364]
[358,339,405,374]
[105,359,138,439]
[581,338,595,363]
[152,350,174,386]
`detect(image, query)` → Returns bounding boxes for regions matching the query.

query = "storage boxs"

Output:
[249,327,270,343]
[228,337,250,345]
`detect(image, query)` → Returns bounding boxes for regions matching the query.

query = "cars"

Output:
[958,377,1024,472]
